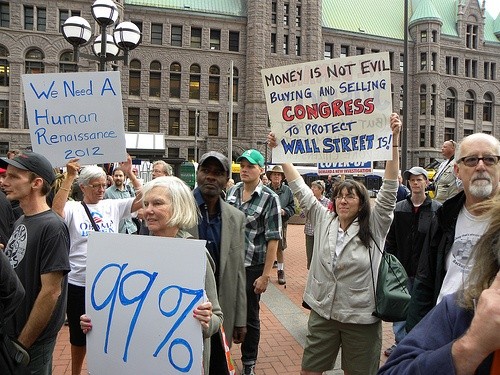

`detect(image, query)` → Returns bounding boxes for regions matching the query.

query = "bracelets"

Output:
[392,144,398,148]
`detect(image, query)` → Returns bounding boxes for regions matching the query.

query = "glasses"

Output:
[334,194,360,201]
[88,184,107,188]
[455,156,500,167]
[316,181,323,188]
[450,140,454,146]
[150,170,166,173]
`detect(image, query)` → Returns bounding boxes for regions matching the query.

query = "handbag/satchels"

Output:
[131,215,141,235]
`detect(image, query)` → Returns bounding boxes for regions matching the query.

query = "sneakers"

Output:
[277,269,286,285]
[273,261,278,268]
[241,361,255,375]
[384,342,399,357]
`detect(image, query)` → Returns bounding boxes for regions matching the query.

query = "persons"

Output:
[405,132,500,333]
[297,179,335,270]
[379,138,462,356]
[265,112,403,375]
[376,193,500,374]
[0,145,297,375]
[323,175,343,200]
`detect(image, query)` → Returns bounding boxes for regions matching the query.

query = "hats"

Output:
[0,151,55,186]
[266,166,286,181]
[331,177,341,181]
[198,151,230,176]
[236,149,264,168]
[403,167,428,180]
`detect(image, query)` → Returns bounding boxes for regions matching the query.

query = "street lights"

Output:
[60,0,141,169]
[256,140,268,174]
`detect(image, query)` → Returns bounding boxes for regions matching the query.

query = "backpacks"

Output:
[369,236,411,322]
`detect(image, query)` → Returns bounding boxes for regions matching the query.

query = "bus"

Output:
[231,163,403,226]
